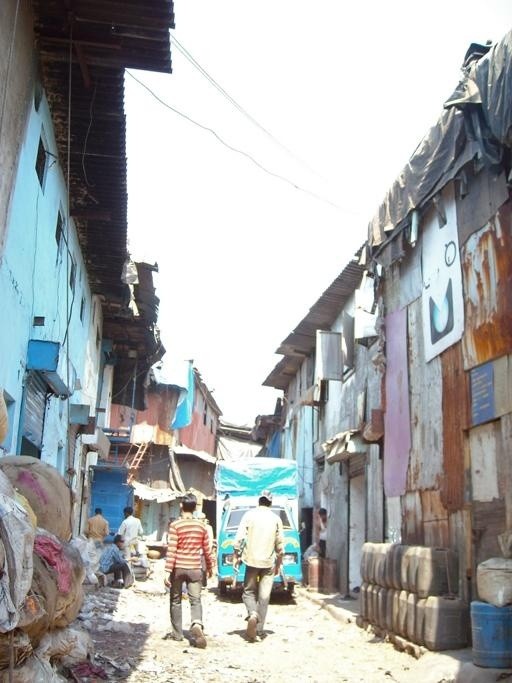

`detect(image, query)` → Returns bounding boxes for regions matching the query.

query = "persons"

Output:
[194,512,213,589]
[100,534,132,589]
[233,489,286,641]
[171,507,188,599]
[163,492,213,649]
[85,508,109,546]
[312,507,327,556]
[118,506,151,585]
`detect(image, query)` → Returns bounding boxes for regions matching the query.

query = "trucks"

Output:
[214,458,302,595]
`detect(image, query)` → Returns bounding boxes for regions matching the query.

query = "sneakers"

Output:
[165,631,182,641]
[190,624,206,648]
[247,616,258,638]
[256,632,266,642]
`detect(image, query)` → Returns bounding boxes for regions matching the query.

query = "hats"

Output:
[260,490,273,502]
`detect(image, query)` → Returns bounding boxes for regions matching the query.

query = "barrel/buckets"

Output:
[470,601,512,668]
[360,542,468,651]
